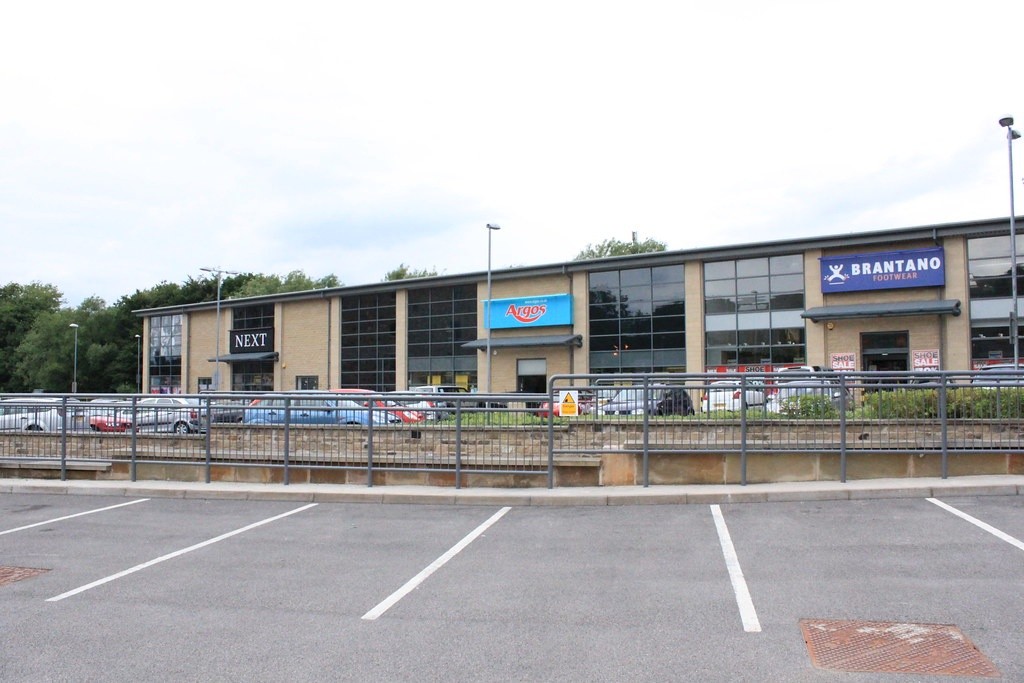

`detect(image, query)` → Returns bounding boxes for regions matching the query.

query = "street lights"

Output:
[70,323,78,392]
[486,224,501,425]
[135,335,141,393]
[999,114,1022,370]
[200,267,238,391]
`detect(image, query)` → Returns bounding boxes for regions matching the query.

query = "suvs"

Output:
[415,386,485,414]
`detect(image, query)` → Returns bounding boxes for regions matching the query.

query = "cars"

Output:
[330,388,425,424]
[702,381,769,412]
[0,397,91,431]
[597,384,695,416]
[388,391,437,420]
[970,363,1023,390]
[210,402,246,423]
[762,381,853,410]
[538,389,595,418]
[592,386,618,406]
[242,390,401,424]
[766,366,850,402]
[909,375,956,389]
[90,398,214,432]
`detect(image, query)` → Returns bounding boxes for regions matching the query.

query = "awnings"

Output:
[206,351,279,363]
[799,299,961,318]
[460,334,582,348]
